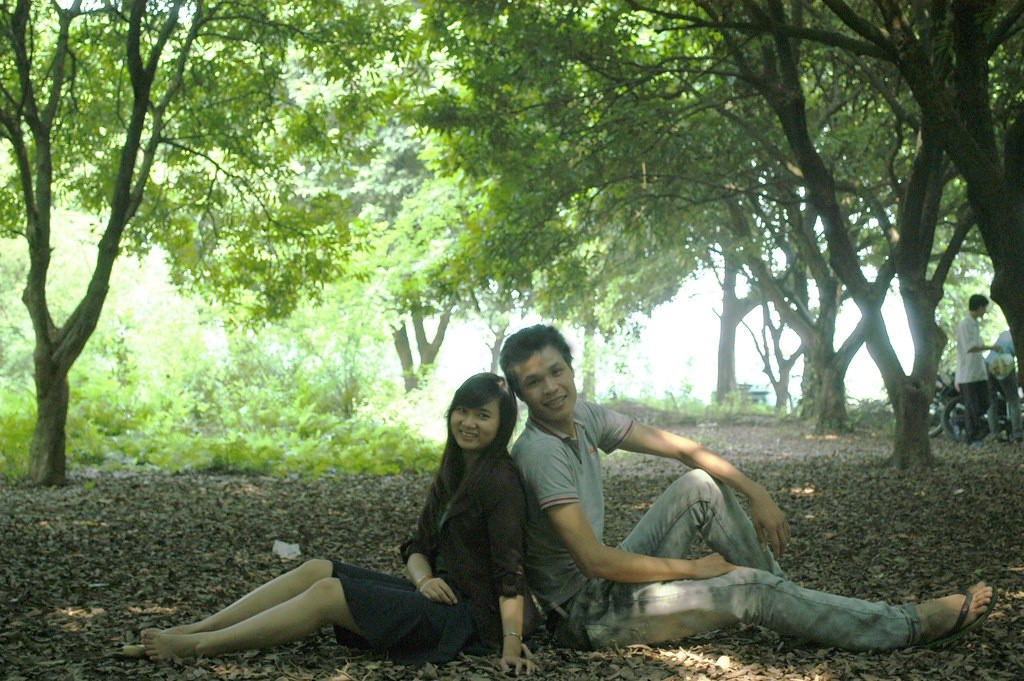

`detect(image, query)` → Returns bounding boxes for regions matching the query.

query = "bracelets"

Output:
[502,633,524,641]
[415,575,437,589]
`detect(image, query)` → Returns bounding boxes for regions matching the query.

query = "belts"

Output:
[545,599,569,633]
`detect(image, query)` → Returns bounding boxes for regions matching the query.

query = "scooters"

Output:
[928,372,1024,442]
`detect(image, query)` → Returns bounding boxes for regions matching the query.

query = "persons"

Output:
[955,294,1024,447]
[140,372,541,676]
[500,324,1000,652]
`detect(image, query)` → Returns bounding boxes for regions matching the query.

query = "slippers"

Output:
[921,580,998,650]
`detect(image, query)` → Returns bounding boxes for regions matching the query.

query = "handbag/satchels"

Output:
[988,349,1014,380]
